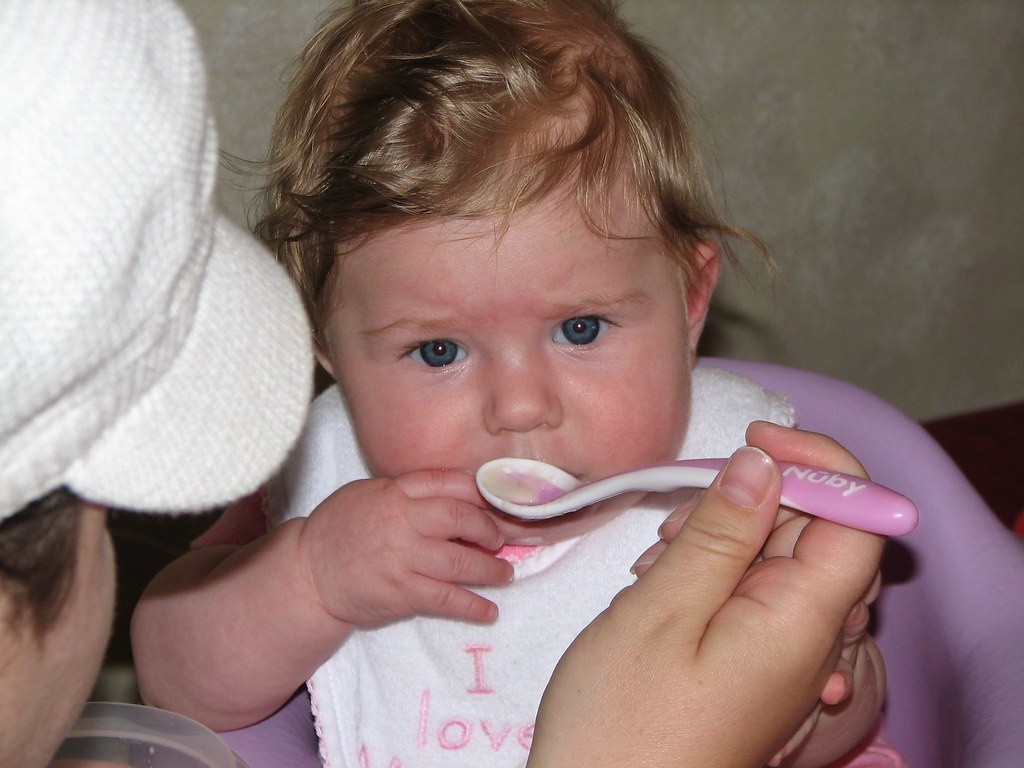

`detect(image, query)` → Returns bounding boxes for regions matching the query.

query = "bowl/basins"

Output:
[41,701,238,768]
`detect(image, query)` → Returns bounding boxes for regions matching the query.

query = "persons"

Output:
[132,1,884,768]
[0,1,887,768]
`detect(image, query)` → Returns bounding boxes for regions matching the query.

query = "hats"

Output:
[0,0,314,523]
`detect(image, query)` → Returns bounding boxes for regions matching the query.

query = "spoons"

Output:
[476,455,919,537]
